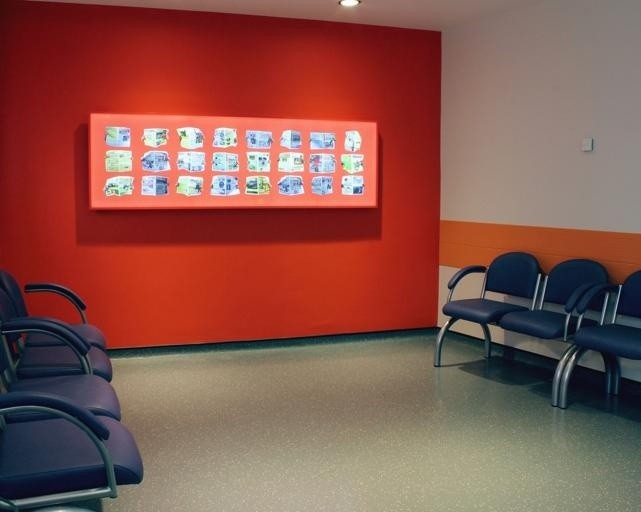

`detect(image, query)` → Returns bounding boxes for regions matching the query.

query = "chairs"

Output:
[0,268,144,512]
[431,252,640,410]
